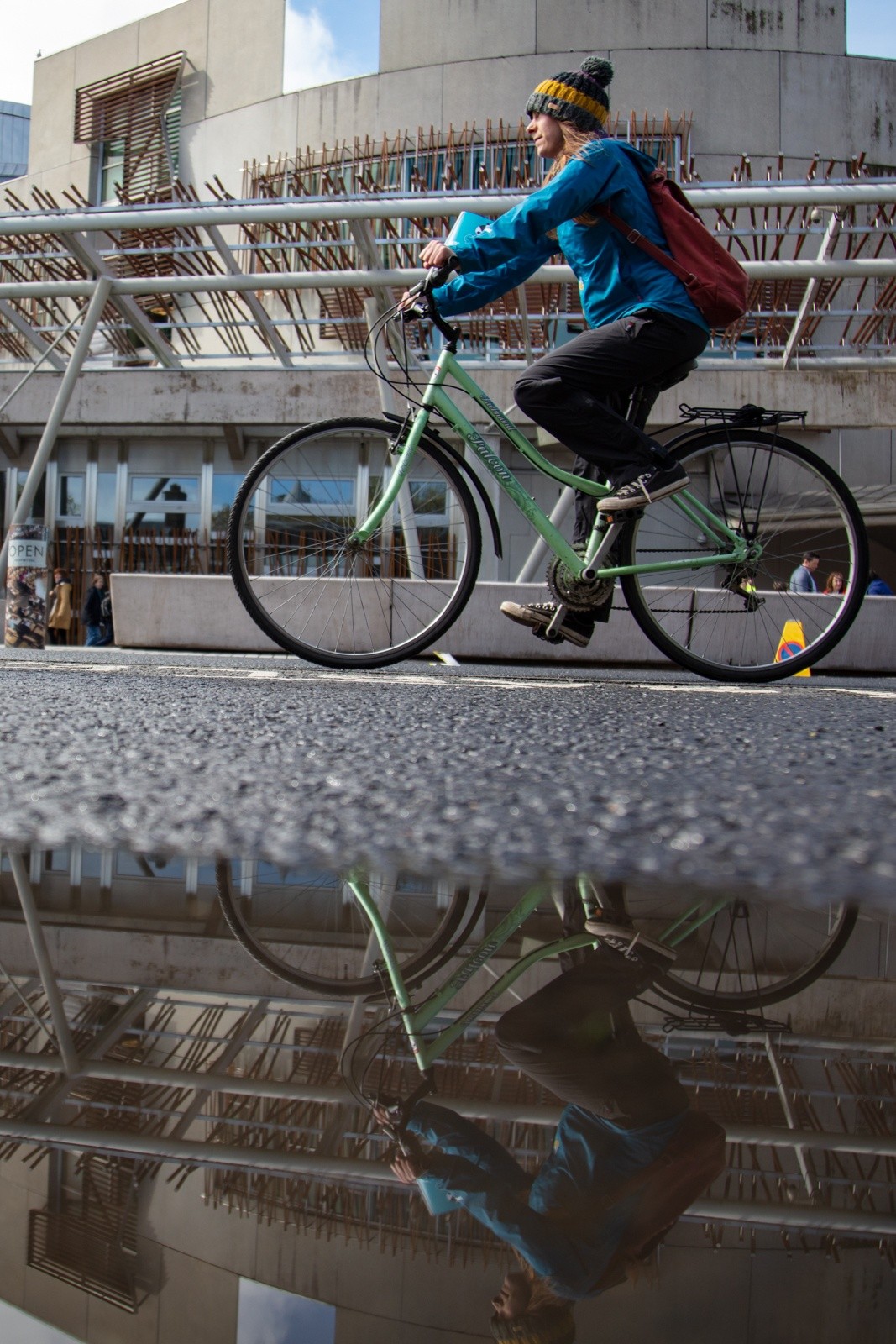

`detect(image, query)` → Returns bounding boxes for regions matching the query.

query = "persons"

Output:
[47,568,109,646]
[792,549,895,596]
[740,570,788,592]
[363,942,738,1343]
[407,54,710,649]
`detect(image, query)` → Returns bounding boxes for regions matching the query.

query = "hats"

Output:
[524,56,612,132]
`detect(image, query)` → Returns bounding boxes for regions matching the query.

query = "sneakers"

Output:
[584,917,679,978]
[577,871,625,954]
[599,460,691,513]
[501,599,596,649]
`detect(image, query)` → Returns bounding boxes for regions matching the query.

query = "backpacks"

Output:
[598,143,750,330]
[551,1122,729,1295]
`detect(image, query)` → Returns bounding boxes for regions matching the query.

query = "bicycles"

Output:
[229,256,871,685]
[212,852,861,1172]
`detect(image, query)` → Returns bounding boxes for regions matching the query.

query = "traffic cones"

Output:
[774,619,811,677]
